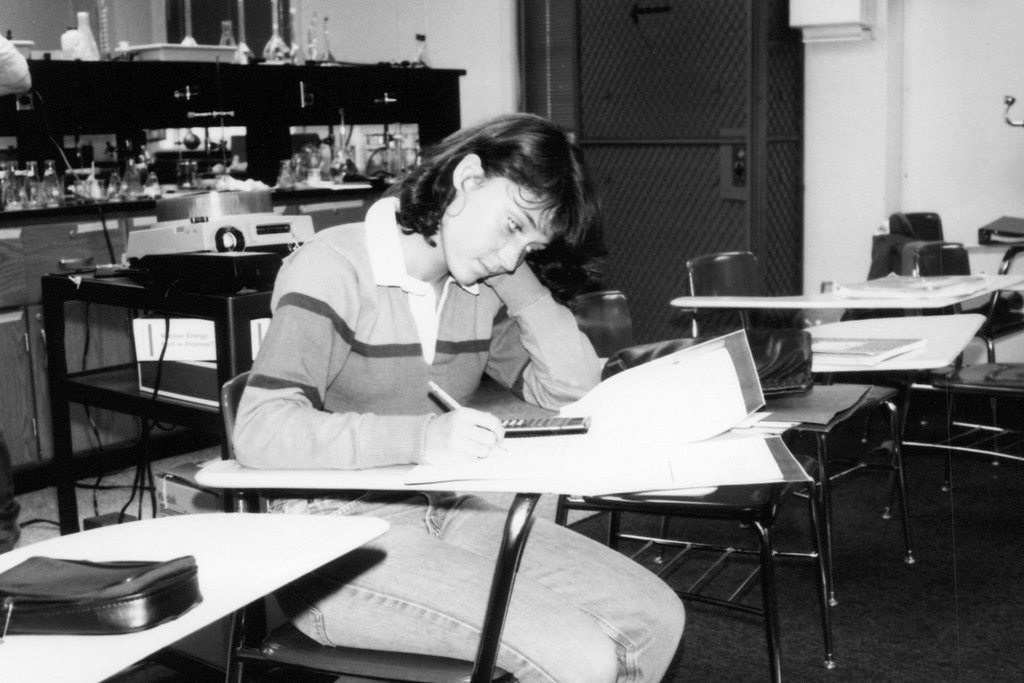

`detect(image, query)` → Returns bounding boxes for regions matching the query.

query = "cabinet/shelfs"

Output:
[41,267,275,537]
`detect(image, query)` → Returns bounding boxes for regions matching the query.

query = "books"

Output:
[809,334,927,366]
[837,274,995,303]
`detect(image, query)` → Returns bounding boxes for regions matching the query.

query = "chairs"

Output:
[554,291,836,683]
[669,213,1023,610]
[220,372,515,683]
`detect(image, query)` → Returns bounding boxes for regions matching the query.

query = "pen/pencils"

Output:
[428,380,462,408]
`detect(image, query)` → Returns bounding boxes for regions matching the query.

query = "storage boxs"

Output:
[127,44,236,63]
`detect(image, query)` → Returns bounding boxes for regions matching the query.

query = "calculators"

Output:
[500,417,590,436]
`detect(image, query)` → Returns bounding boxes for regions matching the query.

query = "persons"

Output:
[0,29,35,553]
[231,110,685,683]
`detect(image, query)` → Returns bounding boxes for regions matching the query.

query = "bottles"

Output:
[304,18,319,60]
[288,10,304,66]
[413,34,429,67]
[73,157,164,206]
[262,0,291,61]
[318,17,337,60]
[16,159,41,212]
[218,21,239,48]
[277,143,360,186]
[38,160,67,208]
[2,159,21,213]
[76,11,100,61]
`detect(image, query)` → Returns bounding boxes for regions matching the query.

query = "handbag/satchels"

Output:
[1,556,202,637]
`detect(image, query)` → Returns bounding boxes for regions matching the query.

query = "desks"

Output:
[672,274,1023,309]
[196,422,721,683]
[796,312,987,371]
[1,512,391,683]
[0,61,467,190]
